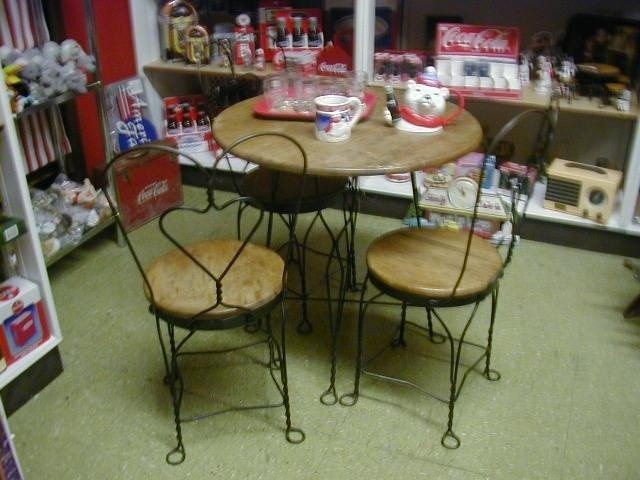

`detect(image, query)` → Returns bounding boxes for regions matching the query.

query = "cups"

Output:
[434,55,523,92]
[261,70,370,112]
[313,96,362,142]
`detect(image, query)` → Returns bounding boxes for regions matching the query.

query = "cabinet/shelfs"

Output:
[132,1,638,261]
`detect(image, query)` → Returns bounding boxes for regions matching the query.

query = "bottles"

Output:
[183,103,194,129]
[166,104,180,134]
[275,15,291,47]
[384,85,400,122]
[196,102,209,131]
[308,16,322,47]
[293,17,308,47]
